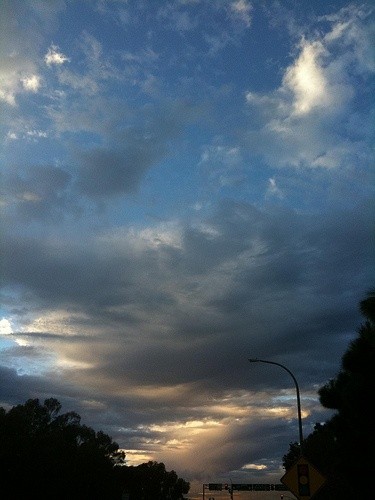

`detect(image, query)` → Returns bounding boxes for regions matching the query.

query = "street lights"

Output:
[248,357,304,455]
